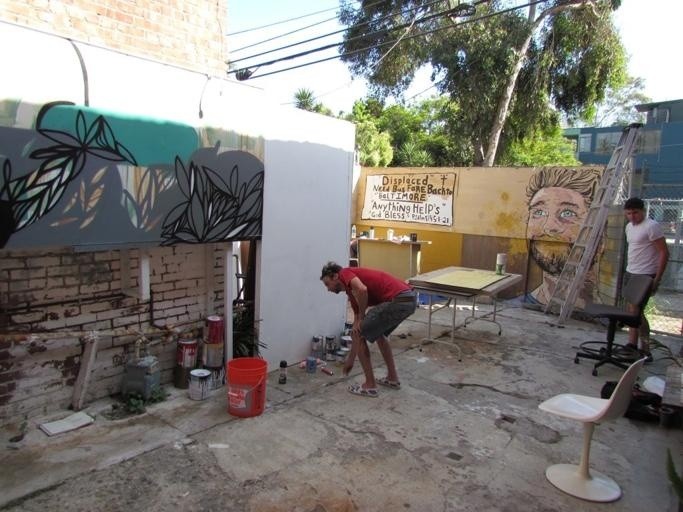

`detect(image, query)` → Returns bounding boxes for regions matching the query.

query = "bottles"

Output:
[370,225,374,239]
[352,225,356,238]
[279,361,287,384]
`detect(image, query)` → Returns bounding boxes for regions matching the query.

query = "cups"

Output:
[306,358,316,373]
[410,233,417,242]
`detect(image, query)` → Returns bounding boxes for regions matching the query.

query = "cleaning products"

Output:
[496,253,507,275]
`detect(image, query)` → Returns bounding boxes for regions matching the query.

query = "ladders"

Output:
[544,123,644,325]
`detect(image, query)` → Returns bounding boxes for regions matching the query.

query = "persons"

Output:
[618,198,669,364]
[317,261,417,399]
[503,165,608,314]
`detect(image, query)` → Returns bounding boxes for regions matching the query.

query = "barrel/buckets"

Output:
[176,316,224,401]
[226,358,266,418]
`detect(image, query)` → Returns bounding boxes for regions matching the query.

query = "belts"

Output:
[397,296,416,301]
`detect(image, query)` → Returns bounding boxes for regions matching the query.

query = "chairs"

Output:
[537,355,649,503]
[574,274,654,381]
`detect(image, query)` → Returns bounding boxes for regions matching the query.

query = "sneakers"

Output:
[346,384,380,397]
[375,376,402,390]
[619,343,653,362]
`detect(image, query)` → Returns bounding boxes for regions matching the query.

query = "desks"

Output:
[404,267,524,362]
[352,237,433,306]
